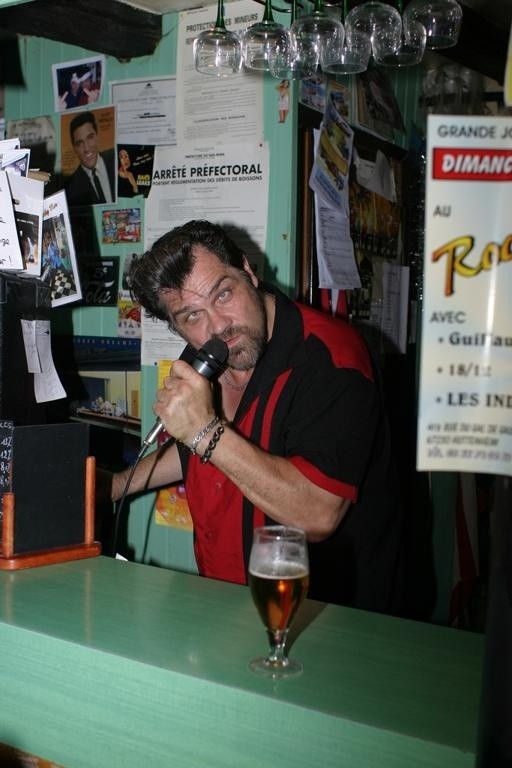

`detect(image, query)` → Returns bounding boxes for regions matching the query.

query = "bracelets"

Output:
[187,413,221,456]
[199,418,229,465]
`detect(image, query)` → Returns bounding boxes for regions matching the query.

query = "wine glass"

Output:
[192,0,463,82]
[247,524,310,677]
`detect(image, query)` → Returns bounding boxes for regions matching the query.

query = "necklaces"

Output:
[221,368,251,393]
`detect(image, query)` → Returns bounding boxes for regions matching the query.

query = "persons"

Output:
[75,70,102,104]
[57,74,84,110]
[125,251,140,287]
[124,299,141,324]
[274,79,291,124]
[61,108,115,205]
[90,396,127,419]
[110,216,430,621]
[40,235,75,290]
[15,211,40,274]
[113,142,141,197]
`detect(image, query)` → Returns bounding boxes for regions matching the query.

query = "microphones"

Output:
[141,335,232,446]
